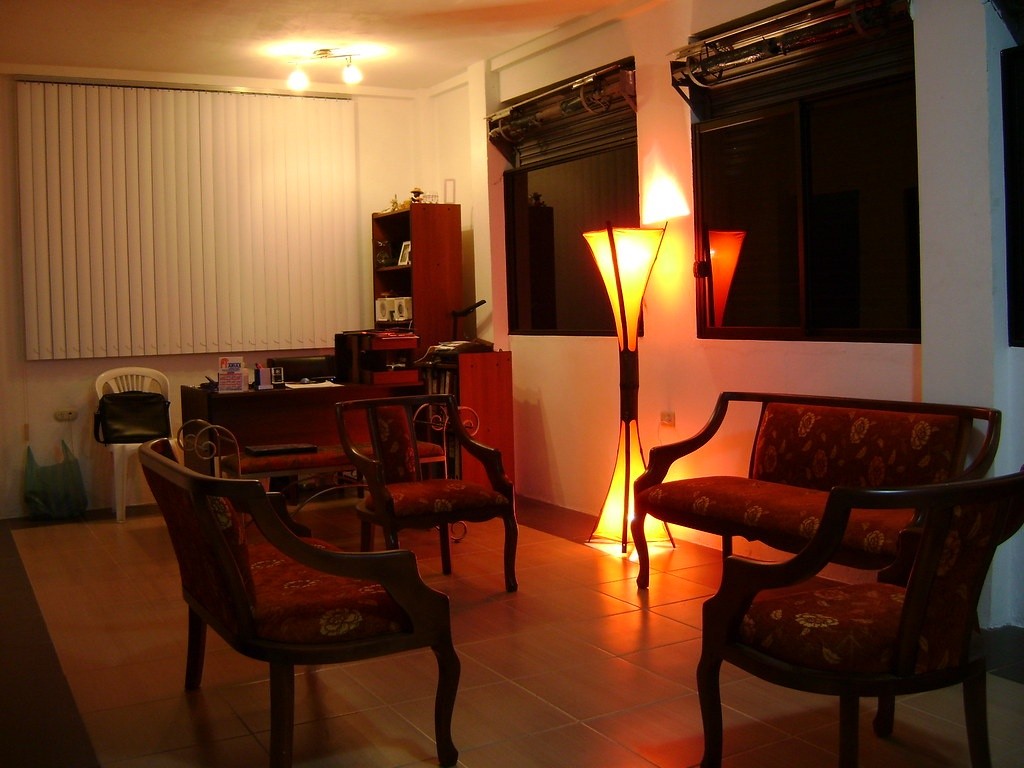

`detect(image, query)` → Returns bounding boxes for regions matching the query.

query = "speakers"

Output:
[375,296,413,322]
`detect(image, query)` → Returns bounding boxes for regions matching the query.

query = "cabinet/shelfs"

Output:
[372,203,465,380]
[424,350,517,514]
[334,330,419,383]
[181,380,427,499]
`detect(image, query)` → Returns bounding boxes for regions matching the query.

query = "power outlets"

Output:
[54,411,78,421]
[661,411,675,428]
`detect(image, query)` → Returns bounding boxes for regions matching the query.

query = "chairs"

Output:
[333,393,518,593]
[95,366,174,523]
[267,355,336,382]
[696,465,1024,768]
[138,437,461,768]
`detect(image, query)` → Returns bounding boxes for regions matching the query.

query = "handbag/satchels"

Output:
[22,440,87,521]
[94,391,170,443]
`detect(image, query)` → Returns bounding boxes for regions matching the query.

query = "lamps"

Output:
[288,49,363,92]
[452,299,488,341]
[580,221,677,554]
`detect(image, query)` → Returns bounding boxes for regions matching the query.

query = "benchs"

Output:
[632,390,1003,590]
[219,442,447,494]
